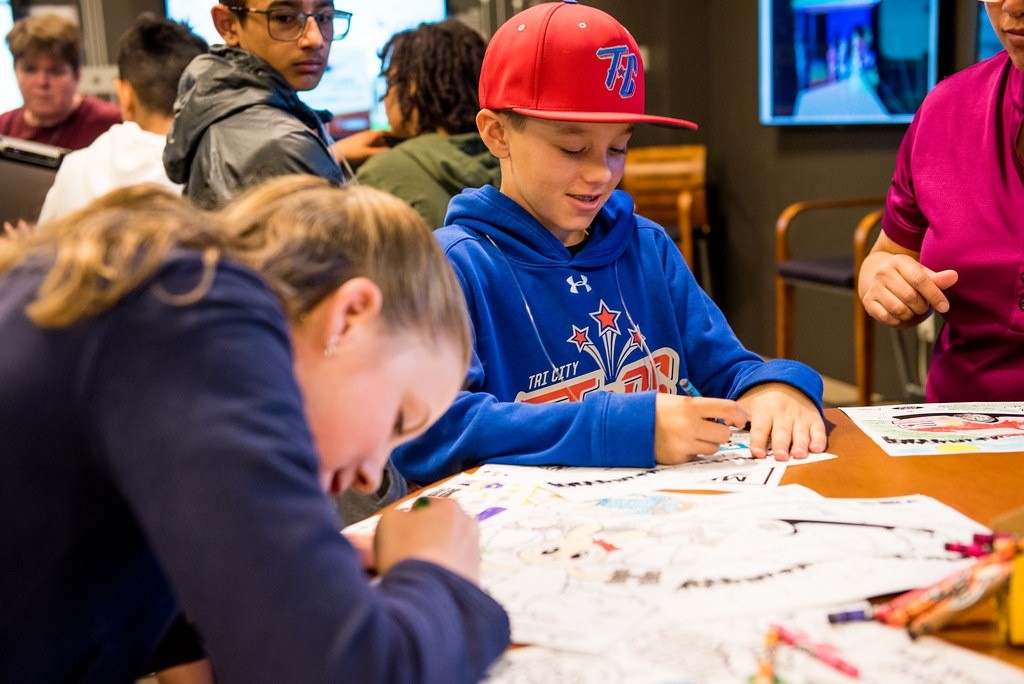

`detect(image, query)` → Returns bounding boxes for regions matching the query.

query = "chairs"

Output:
[622,144,712,295]
[772,196,937,407]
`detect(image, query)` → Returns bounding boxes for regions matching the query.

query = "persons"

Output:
[36,12,208,229]
[327,19,501,230]
[0,5,123,150]
[391,0,828,484]
[163,0,352,211]
[0,171,511,684]
[845,0,1024,403]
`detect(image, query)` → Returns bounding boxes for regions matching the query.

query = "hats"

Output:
[477,0,699,132]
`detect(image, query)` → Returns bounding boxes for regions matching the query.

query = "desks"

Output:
[340,400,1024,683]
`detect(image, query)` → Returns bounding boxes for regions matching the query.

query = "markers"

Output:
[680,379,725,425]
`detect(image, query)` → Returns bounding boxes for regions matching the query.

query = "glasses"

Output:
[376,71,402,102]
[226,5,353,42]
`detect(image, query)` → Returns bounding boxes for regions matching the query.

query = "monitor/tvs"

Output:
[757,0,955,130]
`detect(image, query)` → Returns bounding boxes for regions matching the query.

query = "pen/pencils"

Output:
[772,624,858,677]
[828,609,875,625]
[413,497,428,509]
